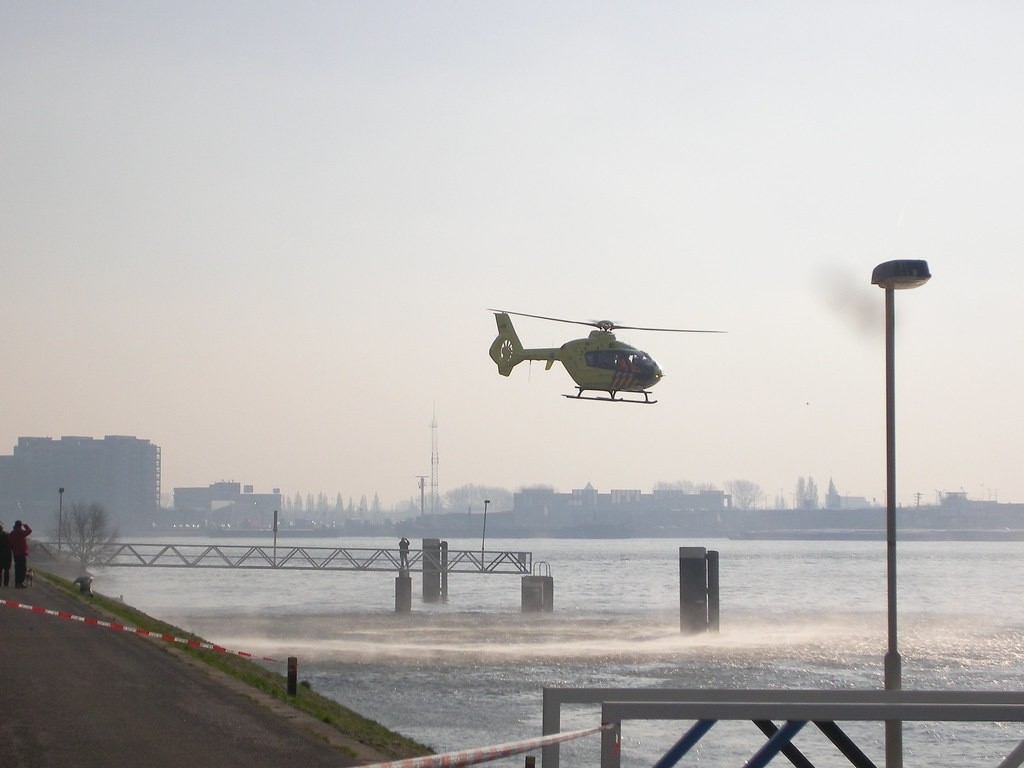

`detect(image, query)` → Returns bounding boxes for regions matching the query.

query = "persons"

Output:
[0,521,12,586]
[8,520,32,588]
[399,538,410,569]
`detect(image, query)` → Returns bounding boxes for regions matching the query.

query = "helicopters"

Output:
[486,309,728,404]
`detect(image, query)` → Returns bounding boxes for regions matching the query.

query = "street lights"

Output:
[482,500,491,571]
[59,487,64,560]
[870,260,932,768]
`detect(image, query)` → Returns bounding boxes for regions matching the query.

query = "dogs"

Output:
[26,568,34,587]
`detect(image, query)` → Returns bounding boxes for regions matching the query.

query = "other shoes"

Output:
[15,583,27,589]
